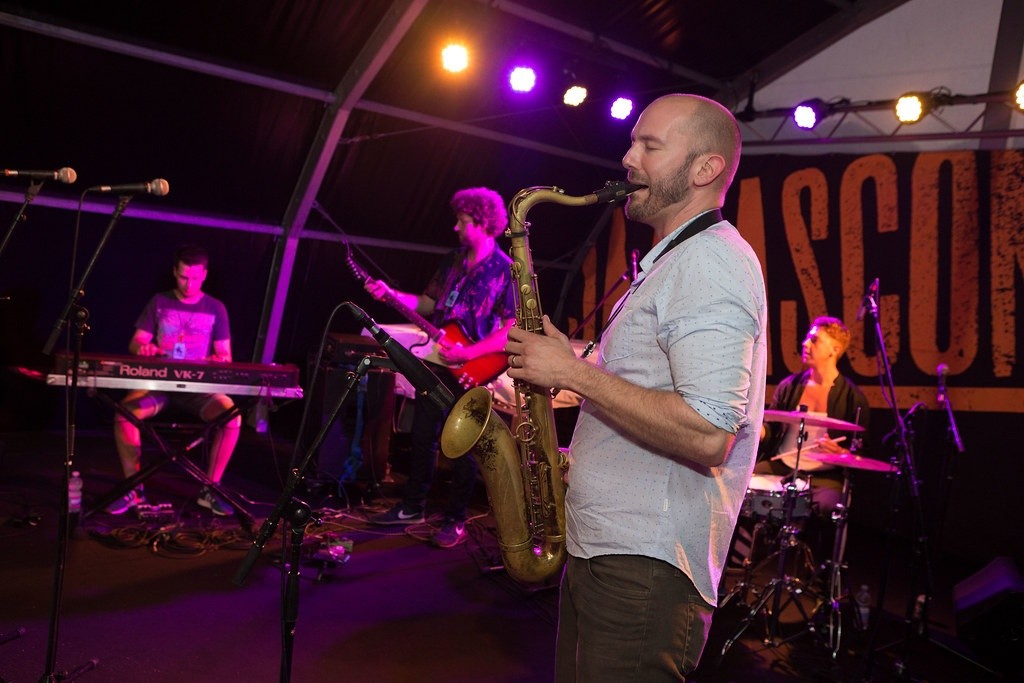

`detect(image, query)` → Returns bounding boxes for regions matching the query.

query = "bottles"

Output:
[67,471,83,514]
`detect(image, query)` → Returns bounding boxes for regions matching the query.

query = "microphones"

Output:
[350,301,455,411]
[856,277,879,321]
[883,403,922,445]
[632,251,637,282]
[936,363,948,407]
[0,167,77,183]
[87,178,169,196]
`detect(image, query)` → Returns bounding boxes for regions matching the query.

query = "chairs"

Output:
[140,410,215,527]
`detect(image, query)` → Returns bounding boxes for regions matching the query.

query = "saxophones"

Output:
[438,180,636,592]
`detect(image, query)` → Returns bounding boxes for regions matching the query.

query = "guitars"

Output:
[342,250,511,391]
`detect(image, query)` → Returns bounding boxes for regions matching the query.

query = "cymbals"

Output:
[802,452,899,472]
[763,409,865,431]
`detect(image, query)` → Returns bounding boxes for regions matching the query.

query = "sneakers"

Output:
[373,503,426,524]
[196,487,234,516]
[431,521,468,548]
[103,482,145,514]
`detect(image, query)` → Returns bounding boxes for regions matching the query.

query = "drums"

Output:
[744,475,813,519]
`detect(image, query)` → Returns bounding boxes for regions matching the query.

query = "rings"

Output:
[511,354,518,367]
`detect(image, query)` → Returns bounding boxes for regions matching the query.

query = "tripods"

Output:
[719,291,1006,681]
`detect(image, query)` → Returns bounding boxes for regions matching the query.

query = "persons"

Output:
[506,94,768,683]
[363,187,520,547]
[103,243,243,517]
[751,316,871,608]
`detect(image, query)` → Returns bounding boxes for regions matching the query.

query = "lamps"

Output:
[790,97,852,130]
[1012,78,1024,113]
[893,92,952,126]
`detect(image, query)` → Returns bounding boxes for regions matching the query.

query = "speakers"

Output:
[306,357,398,503]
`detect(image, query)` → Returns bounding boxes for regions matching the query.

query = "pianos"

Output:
[46,350,305,539]
